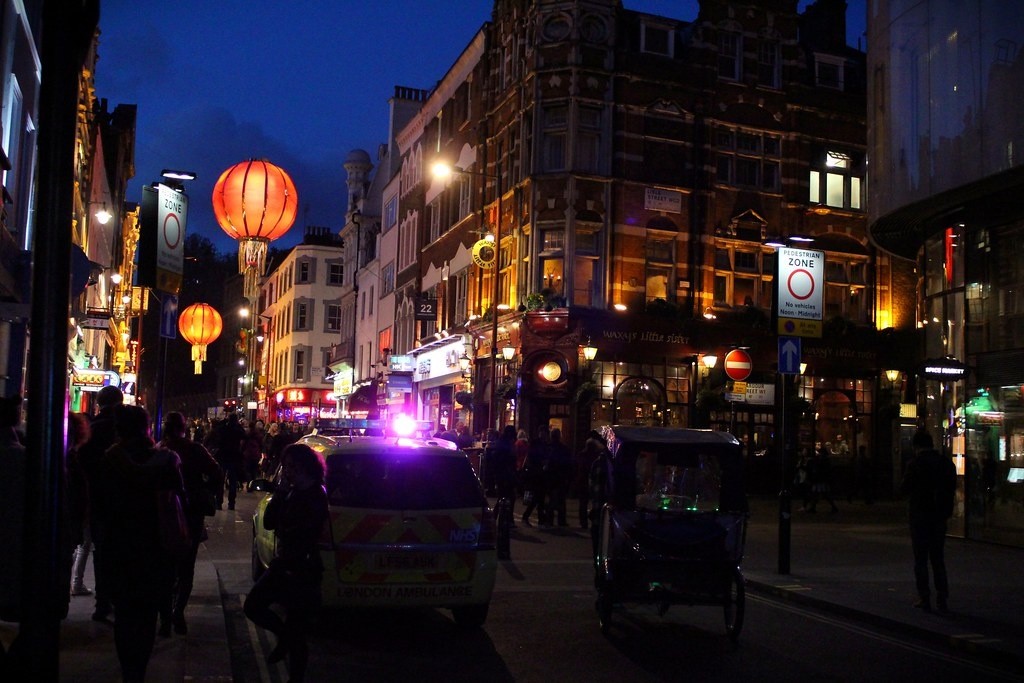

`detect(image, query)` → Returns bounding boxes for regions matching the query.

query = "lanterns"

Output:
[178,303,223,361]
[212,157,298,275]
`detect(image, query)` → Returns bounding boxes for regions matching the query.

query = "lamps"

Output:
[104,266,122,283]
[87,200,112,223]
[110,289,131,303]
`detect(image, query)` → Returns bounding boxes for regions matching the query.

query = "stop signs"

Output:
[724,349,753,382]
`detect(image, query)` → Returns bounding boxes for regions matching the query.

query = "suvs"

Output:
[248,433,498,638]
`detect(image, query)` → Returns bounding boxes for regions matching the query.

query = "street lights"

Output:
[238,307,273,389]
[420,135,503,449]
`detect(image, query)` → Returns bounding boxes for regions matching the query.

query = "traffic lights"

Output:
[223,399,236,410]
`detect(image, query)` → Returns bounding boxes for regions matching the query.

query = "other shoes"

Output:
[92,604,112,621]
[214,503,222,509]
[806,508,816,514]
[830,507,839,515]
[267,643,292,664]
[935,602,947,612]
[228,502,234,509]
[171,611,187,635]
[796,507,806,512]
[521,519,531,527]
[157,621,172,637]
[913,599,932,609]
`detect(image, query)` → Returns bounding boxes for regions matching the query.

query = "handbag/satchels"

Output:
[268,555,318,591]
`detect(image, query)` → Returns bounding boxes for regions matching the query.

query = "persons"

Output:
[886,427,956,609]
[188,407,314,510]
[796,445,840,512]
[0,386,226,683]
[434,422,607,527]
[242,445,329,683]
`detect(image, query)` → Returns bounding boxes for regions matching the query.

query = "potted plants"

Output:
[527,294,544,310]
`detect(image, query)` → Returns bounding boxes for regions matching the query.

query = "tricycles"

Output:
[585,425,751,644]
